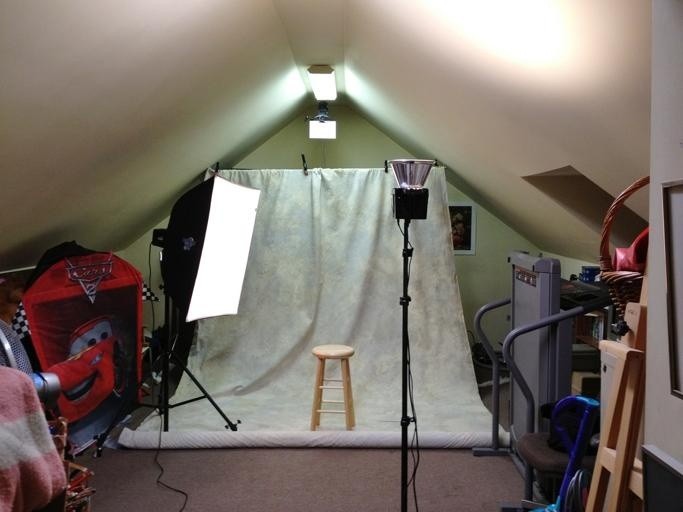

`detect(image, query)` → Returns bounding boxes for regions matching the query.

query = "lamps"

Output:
[308,103,336,139]
[308,66,337,102]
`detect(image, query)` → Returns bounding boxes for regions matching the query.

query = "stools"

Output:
[311,345,355,431]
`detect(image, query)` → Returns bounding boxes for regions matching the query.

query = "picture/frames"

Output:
[449,204,477,255]
